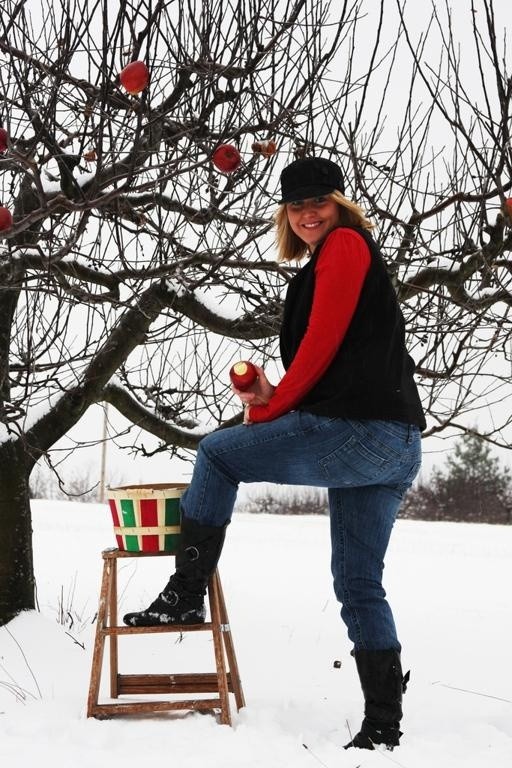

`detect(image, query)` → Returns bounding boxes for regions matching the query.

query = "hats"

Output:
[277,156,346,207]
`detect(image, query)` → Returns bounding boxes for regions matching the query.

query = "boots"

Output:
[341,644,411,752]
[122,508,233,632]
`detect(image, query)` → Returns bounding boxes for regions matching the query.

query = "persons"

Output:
[121,155,429,752]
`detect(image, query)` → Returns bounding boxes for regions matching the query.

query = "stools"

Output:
[87,548,246,726]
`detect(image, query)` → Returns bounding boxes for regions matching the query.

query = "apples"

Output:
[0,128,8,152]
[505,198,512,215]
[119,61,149,93]
[213,144,241,172]
[0,207,12,231]
[260,140,276,157]
[229,361,257,391]
